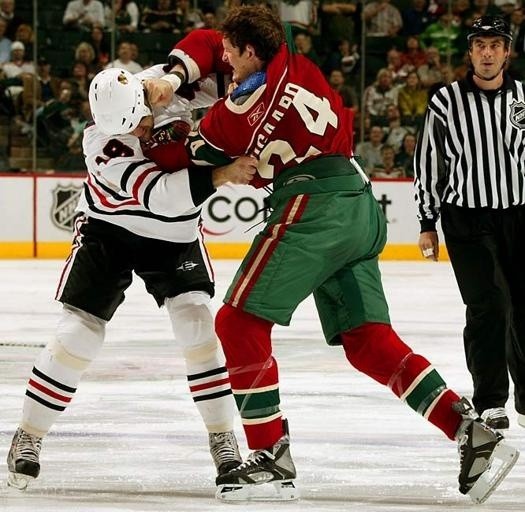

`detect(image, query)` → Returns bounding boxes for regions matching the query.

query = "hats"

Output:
[12,40,26,52]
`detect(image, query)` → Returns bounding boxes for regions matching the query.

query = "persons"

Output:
[394,134,416,176]
[321,0,360,33]
[46,80,74,116]
[0,21,14,63]
[364,69,399,128]
[7,64,259,491]
[353,126,390,172]
[453,51,472,80]
[403,1,435,33]
[76,43,95,65]
[381,103,419,155]
[178,2,201,24]
[330,41,360,71]
[361,0,405,46]
[105,42,143,74]
[288,35,319,65]
[269,0,320,34]
[1,0,23,36]
[426,66,454,105]
[145,7,517,504]
[386,49,412,79]
[71,62,91,101]
[417,47,453,85]
[470,1,488,20]
[105,0,138,31]
[22,56,72,110]
[198,12,218,31]
[494,1,513,19]
[400,35,425,67]
[370,146,406,179]
[2,41,36,107]
[397,71,430,128]
[15,25,36,61]
[140,1,183,33]
[328,69,359,114]
[412,19,525,429]
[87,49,110,86]
[448,1,467,21]
[509,6,525,58]
[88,26,111,66]
[419,8,472,65]
[62,0,107,28]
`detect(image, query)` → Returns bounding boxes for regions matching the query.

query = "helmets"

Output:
[466,15,514,42]
[88,67,151,137]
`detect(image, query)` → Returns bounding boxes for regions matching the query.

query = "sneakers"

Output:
[481,407,509,429]
[215,434,297,487]
[209,431,243,474]
[6,427,43,478]
[458,418,504,495]
